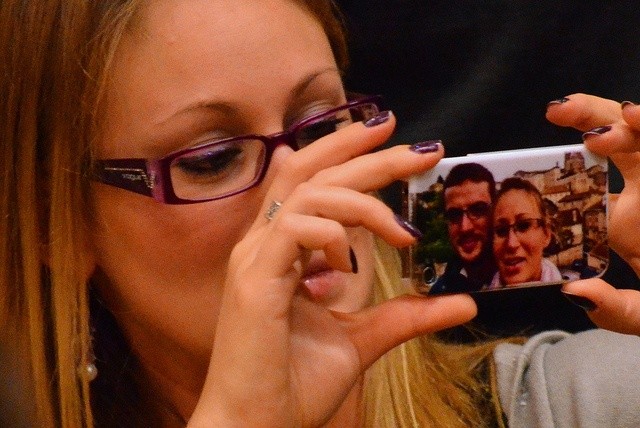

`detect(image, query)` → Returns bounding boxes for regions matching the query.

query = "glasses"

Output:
[493,218,544,237]
[80,92,384,205]
[442,200,493,224]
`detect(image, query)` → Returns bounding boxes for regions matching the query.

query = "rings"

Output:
[265,200,282,221]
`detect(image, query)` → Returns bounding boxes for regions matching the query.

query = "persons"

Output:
[483,177,561,290]
[427,163,496,295]
[0,0,640,428]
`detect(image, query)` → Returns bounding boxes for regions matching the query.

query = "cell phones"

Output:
[400,143,609,298]
